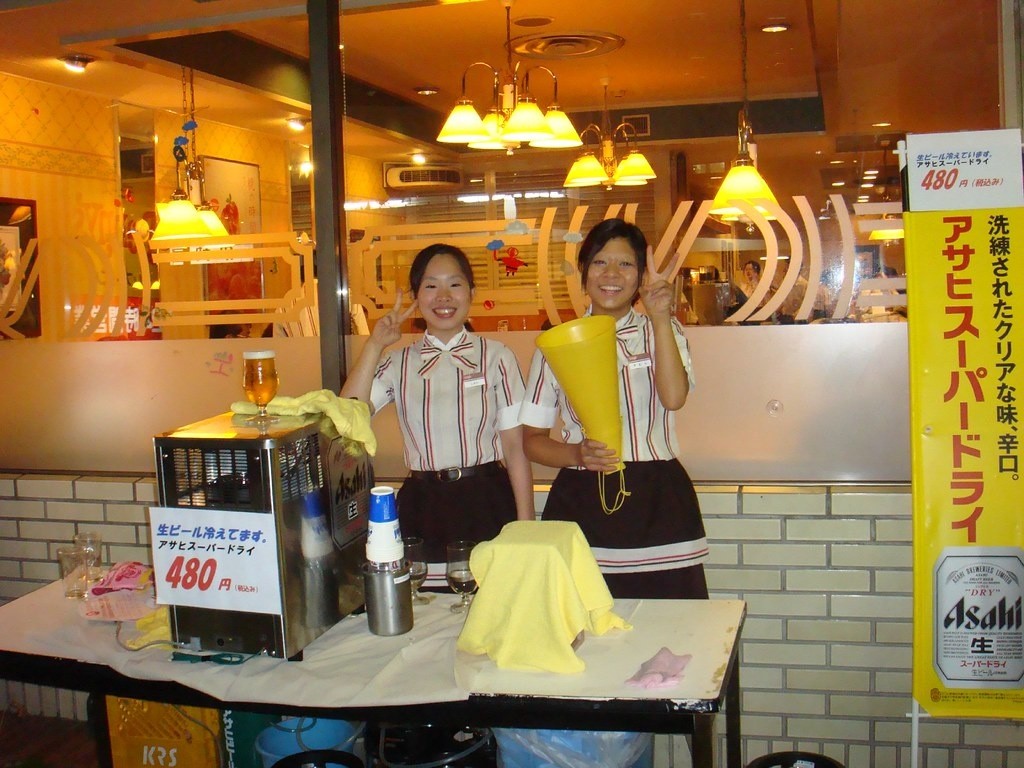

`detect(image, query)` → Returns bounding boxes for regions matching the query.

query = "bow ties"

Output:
[417,333,478,379]
[616,312,641,368]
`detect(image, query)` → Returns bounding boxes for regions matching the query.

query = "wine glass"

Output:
[401,536,430,605]
[445,540,478,614]
[241,350,282,424]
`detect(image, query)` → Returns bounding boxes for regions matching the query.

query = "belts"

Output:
[411,461,504,484]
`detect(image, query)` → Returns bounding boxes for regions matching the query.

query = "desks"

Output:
[0,562,746,768]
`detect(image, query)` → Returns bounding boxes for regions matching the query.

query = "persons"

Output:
[337,244,536,595]
[878,267,907,316]
[519,218,713,751]
[740,261,770,311]
[700,266,747,318]
[771,257,859,325]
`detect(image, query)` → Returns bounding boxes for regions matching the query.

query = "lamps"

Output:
[150,29,233,248]
[867,141,905,247]
[436,0,582,156]
[563,78,658,192]
[64,59,89,73]
[708,0,782,215]
[721,107,778,221]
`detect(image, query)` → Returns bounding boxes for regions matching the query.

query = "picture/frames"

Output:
[855,245,881,280]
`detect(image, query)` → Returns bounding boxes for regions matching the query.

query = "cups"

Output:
[73,532,104,584]
[361,560,414,637]
[56,546,88,600]
[302,491,332,558]
[364,486,405,563]
[301,565,338,627]
[718,272,726,282]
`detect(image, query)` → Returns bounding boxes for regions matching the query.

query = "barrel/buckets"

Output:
[254,716,358,768]
